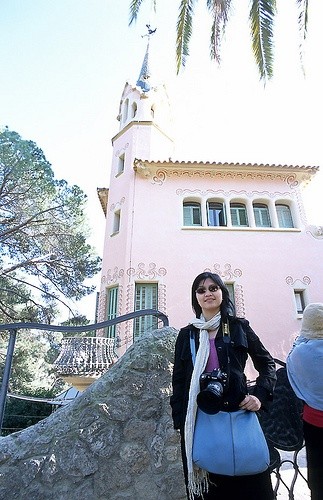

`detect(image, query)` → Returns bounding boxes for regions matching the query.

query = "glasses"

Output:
[195,285,221,294]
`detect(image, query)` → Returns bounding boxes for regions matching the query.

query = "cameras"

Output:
[196,369,232,415]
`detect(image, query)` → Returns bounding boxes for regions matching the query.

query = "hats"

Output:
[299,303,323,339]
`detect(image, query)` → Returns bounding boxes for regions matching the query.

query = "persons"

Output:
[287,304,323,500]
[168,272,278,500]
